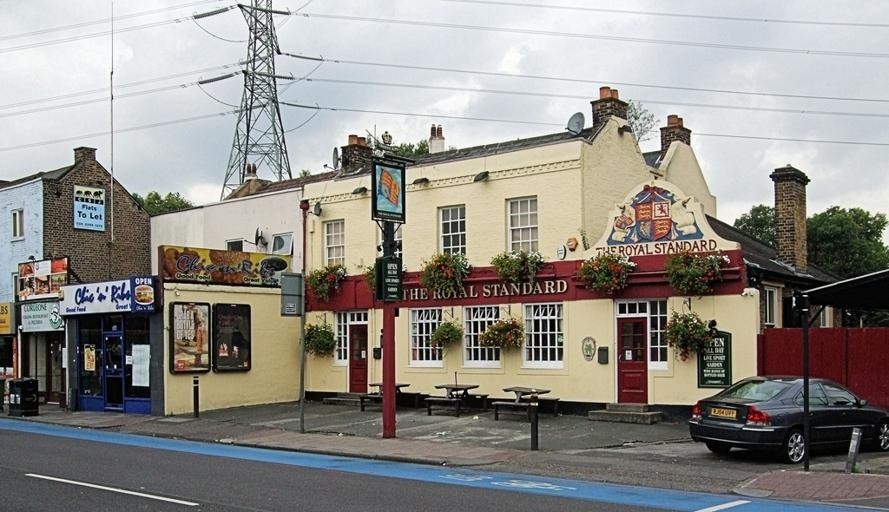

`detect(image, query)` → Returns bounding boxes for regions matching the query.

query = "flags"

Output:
[377,169,400,208]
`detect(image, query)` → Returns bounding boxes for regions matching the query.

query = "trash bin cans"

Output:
[7,376,39,417]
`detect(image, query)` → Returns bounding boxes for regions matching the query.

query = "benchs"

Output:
[492,401,531,422]
[522,396,561,418]
[452,393,489,411]
[424,397,462,417]
[396,391,420,409]
[360,394,383,411]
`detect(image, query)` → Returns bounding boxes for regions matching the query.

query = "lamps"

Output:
[617,125,633,136]
[412,177,431,185]
[473,171,490,183]
[351,187,367,196]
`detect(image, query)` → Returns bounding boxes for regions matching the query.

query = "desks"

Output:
[368,382,410,396]
[435,383,479,411]
[502,386,551,403]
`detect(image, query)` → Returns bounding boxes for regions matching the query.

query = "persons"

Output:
[193,313,205,367]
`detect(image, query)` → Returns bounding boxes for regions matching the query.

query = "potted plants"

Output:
[416,250,472,291]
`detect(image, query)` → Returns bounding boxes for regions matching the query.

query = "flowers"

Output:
[490,248,545,286]
[357,262,408,295]
[665,249,731,297]
[664,307,712,362]
[425,314,526,359]
[303,322,336,360]
[579,248,638,298]
[304,264,349,303]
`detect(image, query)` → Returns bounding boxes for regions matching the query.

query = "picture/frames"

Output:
[169,302,251,374]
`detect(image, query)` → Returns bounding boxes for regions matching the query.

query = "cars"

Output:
[688,375,889,463]
